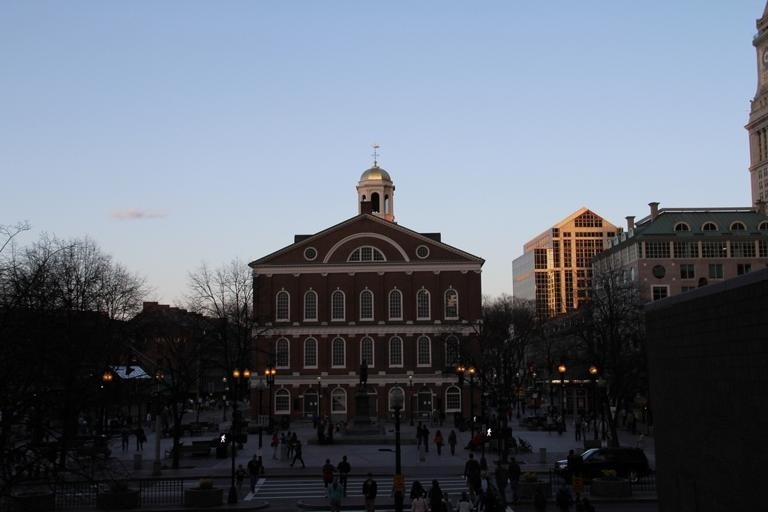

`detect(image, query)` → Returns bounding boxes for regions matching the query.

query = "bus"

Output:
[527,378,604,415]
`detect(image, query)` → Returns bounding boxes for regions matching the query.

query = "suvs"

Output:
[553,447,651,484]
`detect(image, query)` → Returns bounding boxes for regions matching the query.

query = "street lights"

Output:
[222,376,227,396]
[457,367,465,420]
[557,364,568,418]
[233,368,251,401]
[316,375,323,416]
[222,394,227,422]
[532,371,538,417]
[407,375,416,425]
[515,372,521,417]
[467,367,476,438]
[101,371,114,423]
[264,363,277,429]
[195,396,203,423]
[155,374,164,391]
[588,365,598,439]
[389,388,404,476]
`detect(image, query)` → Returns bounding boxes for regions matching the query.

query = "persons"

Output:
[235,464,247,490]
[410,399,527,512]
[248,454,259,494]
[392,471,405,512]
[322,459,337,498]
[359,357,367,384]
[362,473,377,512]
[543,405,646,512]
[135,424,147,451]
[328,481,344,512]
[270,411,351,467]
[120,428,131,456]
[337,455,350,498]
